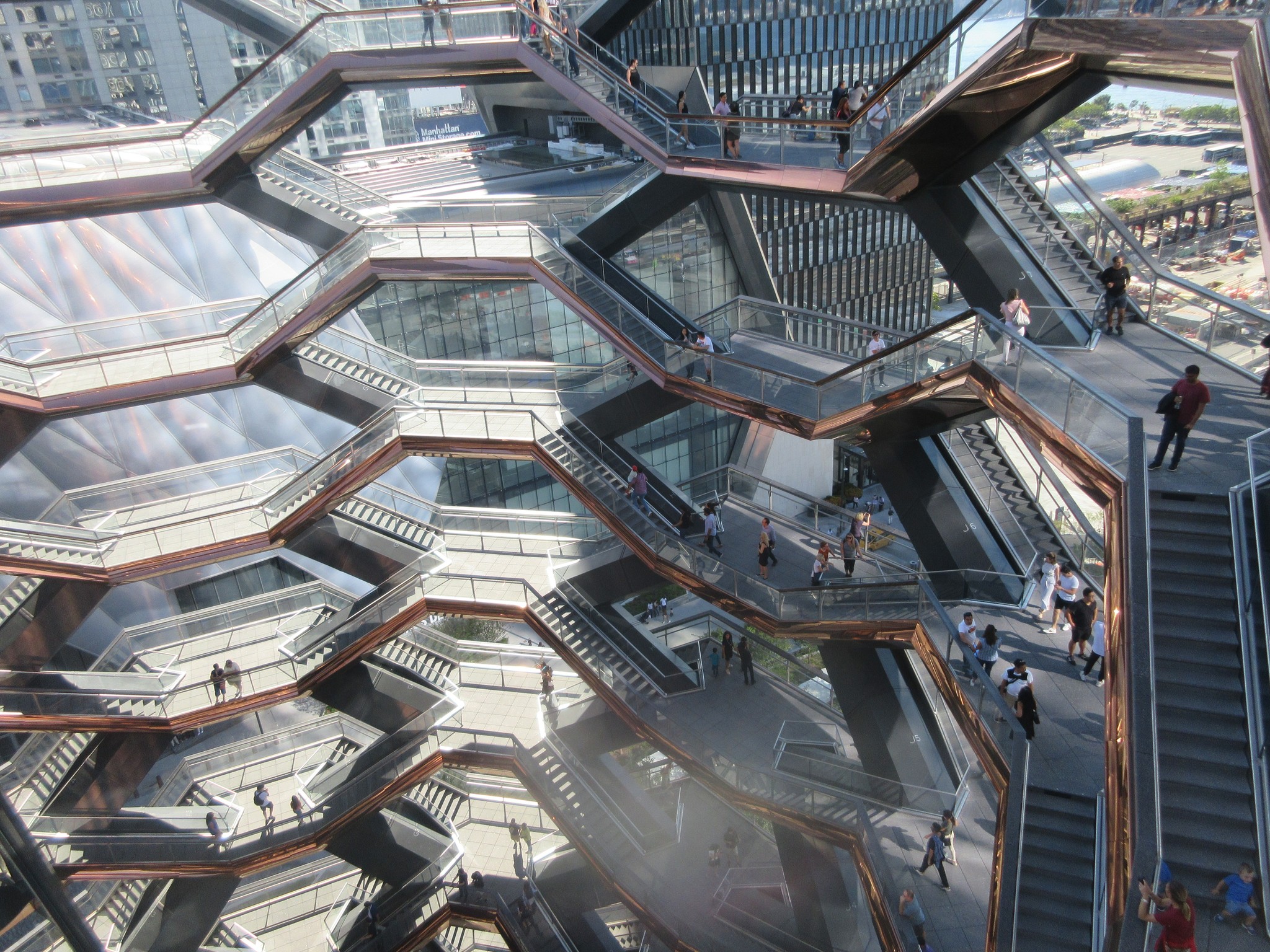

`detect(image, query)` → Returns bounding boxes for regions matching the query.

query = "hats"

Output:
[632,466,637,471]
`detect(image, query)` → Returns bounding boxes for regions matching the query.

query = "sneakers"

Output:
[1037,610,1104,687]
[970,679,1009,723]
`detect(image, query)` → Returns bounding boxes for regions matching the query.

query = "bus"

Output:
[1132,130,1212,146]
[1233,145,1245,158]
[1203,144,1237,162]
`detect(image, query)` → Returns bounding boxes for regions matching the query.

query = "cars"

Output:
[1165,111,1180,119]
[1162,123,1177,128]
[1153,121,1165,126]
[1105,118,1129,126]
[1184,120,1199,125]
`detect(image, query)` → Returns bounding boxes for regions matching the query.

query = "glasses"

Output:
[874,333,880,336]
[846,537,852,539]
[1019,660,1025,663]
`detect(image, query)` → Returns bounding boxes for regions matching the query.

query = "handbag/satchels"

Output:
[998,685,1006,693]
[782,111,789,117]
[1012,307,1030,326]
[813,572,822,581]
[944,838,952,846]
[973,643,978,657]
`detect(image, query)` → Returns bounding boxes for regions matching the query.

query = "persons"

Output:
[1147,364,1210,471]
[673,493,894,586]
[827,80,939,169]
[626,360,638,382]
[448,818,538,934]
[171,661,302,861]
[652,763,675,809]
[641,595,757,685]
[1100,256,1131,334]
[364,900,380,939]
[673,325,713,388]
[1000,286,1029,366]
[959,612,1041,742]
[1138,861,1259,952]
[627,58,643,120]
[675,90,695,149]
[626,465,652,517]
[1127,0,1265,18]
[528,639,554,700]
[421,0,454,47]
[900,805,956,951]
[1034,551,1107,688]
[868,330,954,390]
[505,0,581,79]
[707,826,741,879]
[787,94,810,140]
[713,92,743,160]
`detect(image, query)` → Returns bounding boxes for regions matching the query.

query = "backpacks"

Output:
[253,790,265,805]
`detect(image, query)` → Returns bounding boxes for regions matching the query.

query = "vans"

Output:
[859,527,896,552]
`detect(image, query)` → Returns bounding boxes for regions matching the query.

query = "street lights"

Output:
[1157,229,1166,263]
[1235,273,1244,298]
[840,453,848,534]
[1229,209,1241,238]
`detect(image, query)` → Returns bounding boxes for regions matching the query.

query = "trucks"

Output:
[1074,139,1093,154]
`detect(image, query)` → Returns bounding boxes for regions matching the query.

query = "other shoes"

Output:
[1106,325,1124,335]
[713,664,756,685]
[625,490,853,599]
[1242,922,1259,935]
[998,360,1021,367]
[1147,462,1178,471]
[673,136,848,169]
[1214,913,1225,923]
[522,32,644,120]
[912,845,957,891]
[221,815,304,847]
[870,383,889,390]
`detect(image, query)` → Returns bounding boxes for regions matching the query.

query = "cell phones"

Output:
[1138,875,1146,884]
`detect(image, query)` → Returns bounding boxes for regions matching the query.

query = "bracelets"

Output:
[1140,898,1148,904]
[833,555,835,556]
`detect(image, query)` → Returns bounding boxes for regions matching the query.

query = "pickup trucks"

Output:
[1017,156,1033,165]
